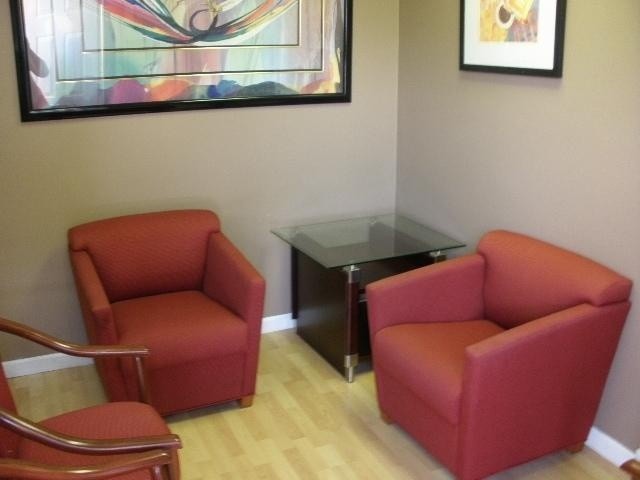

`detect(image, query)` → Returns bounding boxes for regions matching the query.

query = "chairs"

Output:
[362,230,634,480]
[67,205,266,425]
[0,314,184,479]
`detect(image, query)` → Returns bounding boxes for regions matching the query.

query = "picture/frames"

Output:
[457,0,570,79]
[7,0,352,122]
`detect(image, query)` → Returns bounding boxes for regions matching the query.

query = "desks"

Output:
[265,212,467,385]
